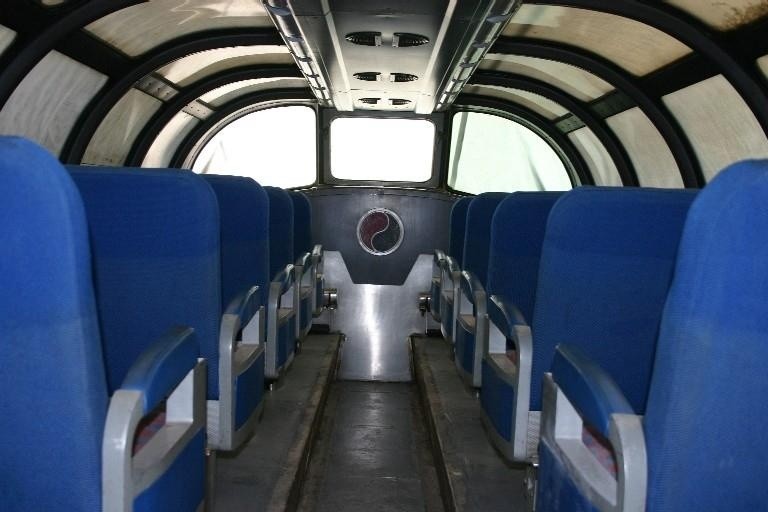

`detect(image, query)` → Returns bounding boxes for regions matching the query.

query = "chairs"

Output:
[1,130,322,511]
[430,157,768,511]
